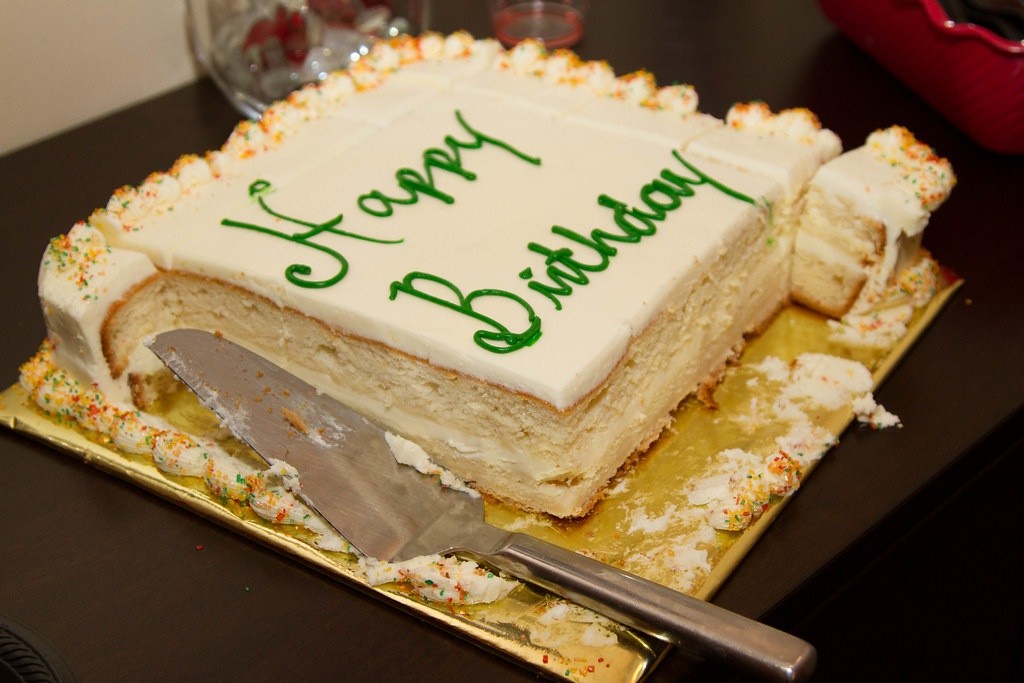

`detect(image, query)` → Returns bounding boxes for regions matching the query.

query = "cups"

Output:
[482,0,588,50]
[185,0,426,121]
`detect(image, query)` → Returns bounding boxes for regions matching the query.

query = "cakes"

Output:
[36,30,953,525]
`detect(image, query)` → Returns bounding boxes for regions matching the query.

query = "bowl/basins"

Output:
[823,0,1024,157]
